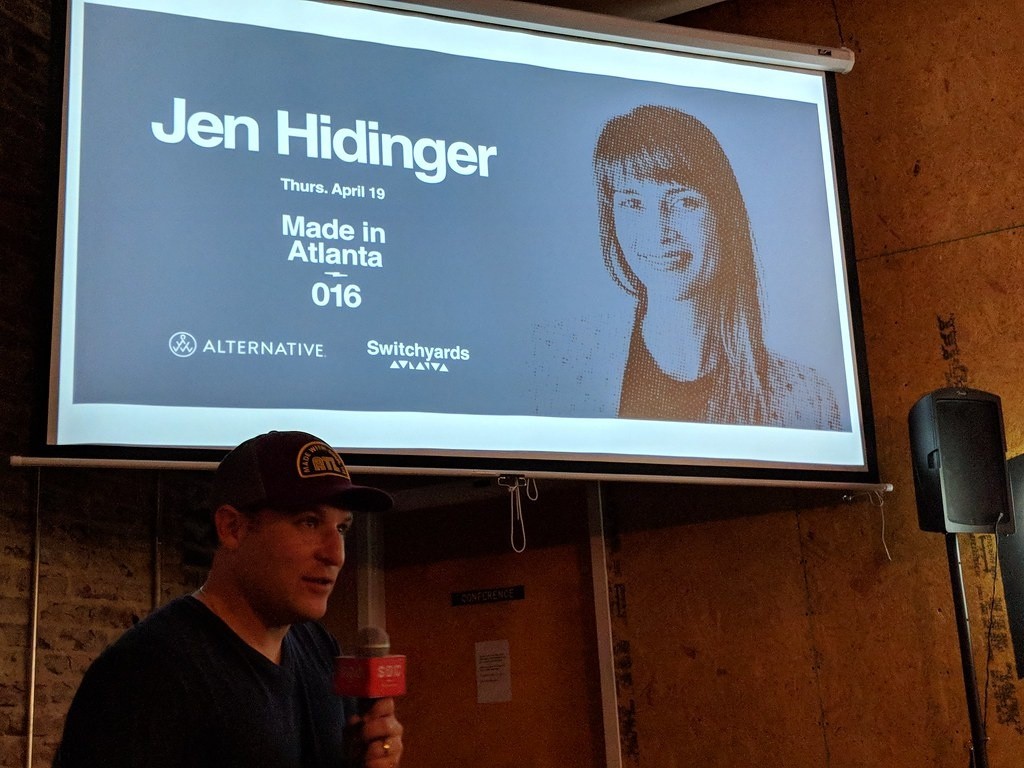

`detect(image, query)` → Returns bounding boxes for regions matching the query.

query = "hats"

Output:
[209,430,394,511]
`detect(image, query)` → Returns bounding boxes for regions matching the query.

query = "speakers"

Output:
[909,386,1015,536]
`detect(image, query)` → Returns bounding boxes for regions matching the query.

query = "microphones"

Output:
[330,623,406,752]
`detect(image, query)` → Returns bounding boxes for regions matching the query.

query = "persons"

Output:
[43,428,409,768]
[532,102,846,437]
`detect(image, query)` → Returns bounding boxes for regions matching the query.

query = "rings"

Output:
[381,737,392,758]
